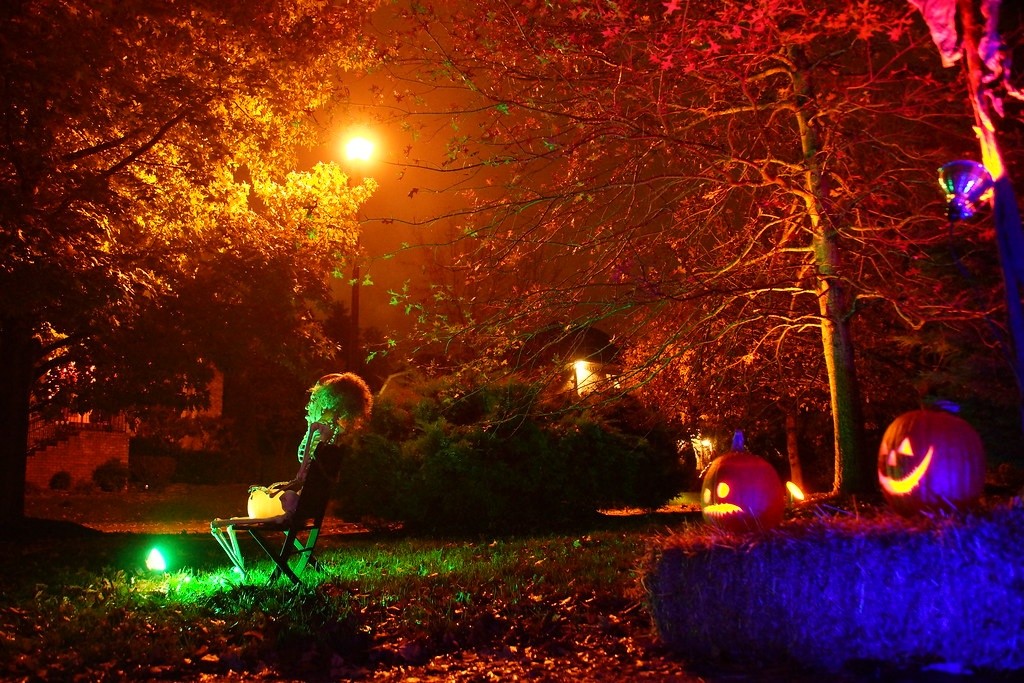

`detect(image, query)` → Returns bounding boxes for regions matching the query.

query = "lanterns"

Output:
[701,451,784,531]
[878,410,985,517]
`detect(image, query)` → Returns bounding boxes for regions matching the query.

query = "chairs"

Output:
[233,441,346,585]
